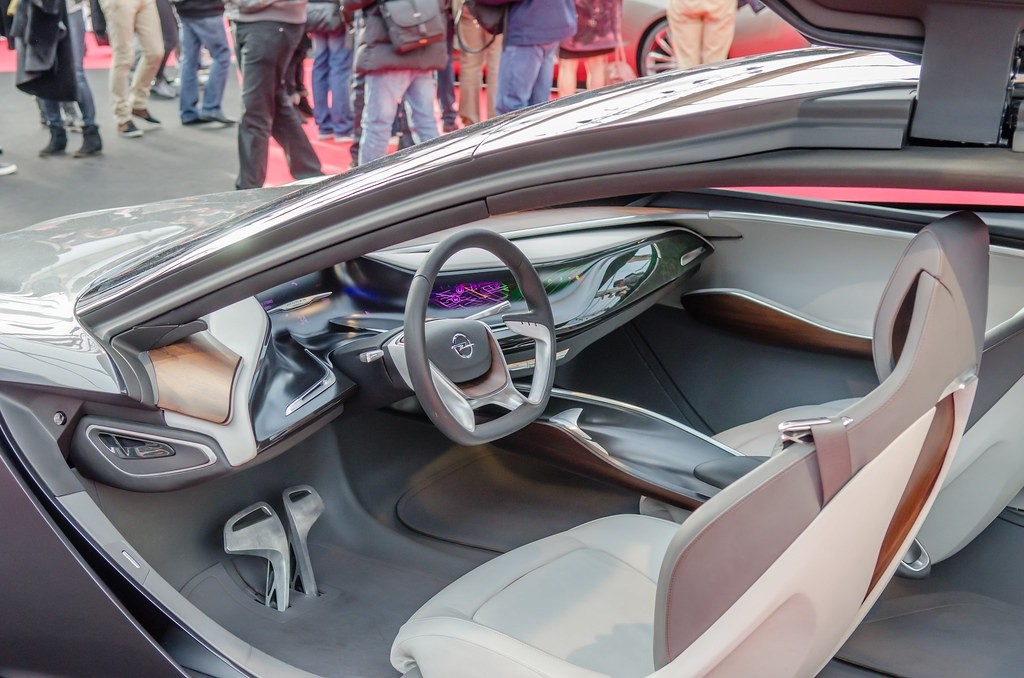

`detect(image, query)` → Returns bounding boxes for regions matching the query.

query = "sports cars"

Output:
[0,0,1024,678]
[450,0,928,91]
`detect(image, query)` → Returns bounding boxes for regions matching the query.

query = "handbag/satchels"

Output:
[455,0,505,55]
[379,0,444,56]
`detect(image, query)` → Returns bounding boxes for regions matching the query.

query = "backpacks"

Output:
[605,45,635,88]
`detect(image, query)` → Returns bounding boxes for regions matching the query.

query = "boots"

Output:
[39,125,66,157]
[73,125,101,158]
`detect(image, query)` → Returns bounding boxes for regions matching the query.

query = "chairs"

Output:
[387,211,993,678]
[714,304,1024,564]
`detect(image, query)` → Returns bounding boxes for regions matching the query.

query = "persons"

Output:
[665,0,736,69]
[613,273,645,298]
[0,0,624,192]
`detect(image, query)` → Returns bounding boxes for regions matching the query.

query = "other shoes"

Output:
[0,162,17,175]
[133,110,161,126]
[118,120,143,137]
[201,106,237,123]
[317,128,335,139]
[332,132,355,142]
[295,97,313,123]
[182,112,213,123]
[169,78,180,95]
[443,122,458,133]
[152,78,176,98]
